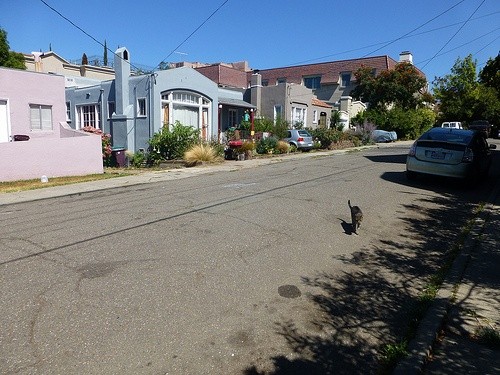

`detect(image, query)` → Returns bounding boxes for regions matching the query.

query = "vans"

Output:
[440,121,463,129]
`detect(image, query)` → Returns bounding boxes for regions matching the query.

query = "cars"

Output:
[405,128,489,192]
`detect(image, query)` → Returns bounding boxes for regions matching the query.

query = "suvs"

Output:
[280,129,315,152]
[469,120,492,136]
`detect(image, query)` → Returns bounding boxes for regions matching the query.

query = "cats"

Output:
[348,200,363,235]
